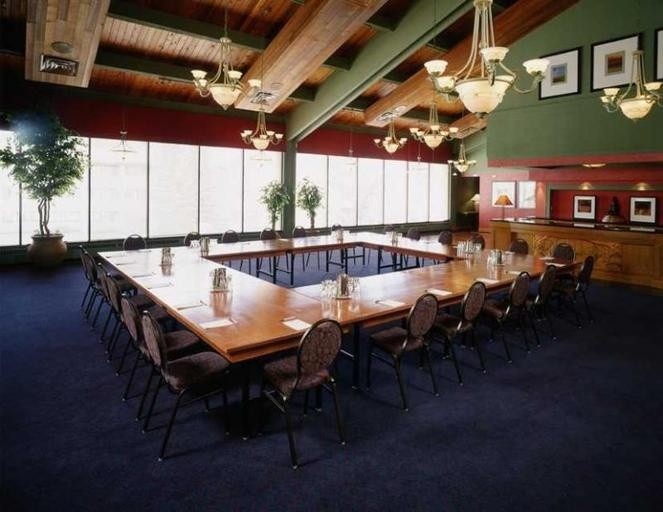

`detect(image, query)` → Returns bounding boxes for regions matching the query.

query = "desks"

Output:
[457,210,479,230]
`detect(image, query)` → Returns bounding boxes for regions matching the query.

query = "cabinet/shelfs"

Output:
[485,217,663,295]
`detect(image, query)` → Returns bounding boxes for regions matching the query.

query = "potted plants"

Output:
[297,179,321,235]
[259,182,291,238]
[0,104,94,273]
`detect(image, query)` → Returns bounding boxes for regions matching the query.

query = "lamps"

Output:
[113,100,139,163]
[189,0,263,111]
[600,42,663,125]
[239,91,284,153]
[576,182,595,191]
[424,0,551,119]
[373,75,475,171]
[632,183,658,190]
[494,194,512,219]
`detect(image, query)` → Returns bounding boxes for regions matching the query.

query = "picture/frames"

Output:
[627,195,657,229]
[536,45,583,99]
[490,180,517,209]
[654,27,663,80]
[590,30,642,94]
[571,195,596,223]
